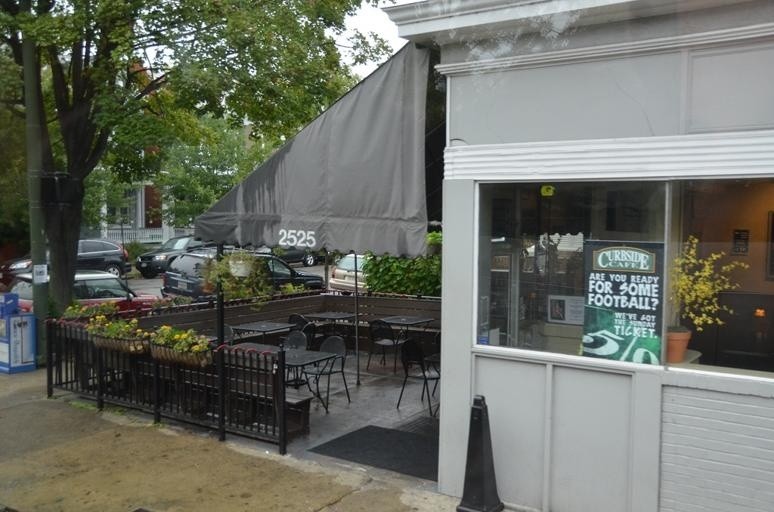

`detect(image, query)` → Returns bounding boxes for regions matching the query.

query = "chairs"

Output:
[223,312,441,416]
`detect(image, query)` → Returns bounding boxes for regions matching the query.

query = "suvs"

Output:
[156,242,324,301]
[7,268,162,321]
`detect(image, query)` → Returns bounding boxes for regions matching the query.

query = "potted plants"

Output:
[667,234,751,363]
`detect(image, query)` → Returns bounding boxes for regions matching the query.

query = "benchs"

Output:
[269,389,314,441]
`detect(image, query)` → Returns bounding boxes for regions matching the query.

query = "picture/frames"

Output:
[730,229,750,255]
[764,211,774,281]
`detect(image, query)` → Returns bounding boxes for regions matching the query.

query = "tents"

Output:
[194,40,442,444]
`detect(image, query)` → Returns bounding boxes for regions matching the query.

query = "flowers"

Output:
[150,324,211,353]
[87,315,150,340]
[63,300,120,319]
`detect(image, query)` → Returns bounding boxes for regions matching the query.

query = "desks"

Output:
[197,334,218,341]
[537,321,583,355]
[684,350,702,363]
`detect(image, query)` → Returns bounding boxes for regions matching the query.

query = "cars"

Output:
[135,234,216,279]
[245,242,335,267]
[327,254,372,296]
[0,238,133,288]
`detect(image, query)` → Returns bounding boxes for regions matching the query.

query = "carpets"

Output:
[305,425,438,482]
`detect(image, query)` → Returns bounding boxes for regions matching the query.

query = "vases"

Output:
[150,345,212,368]
[92,333,146,356]
